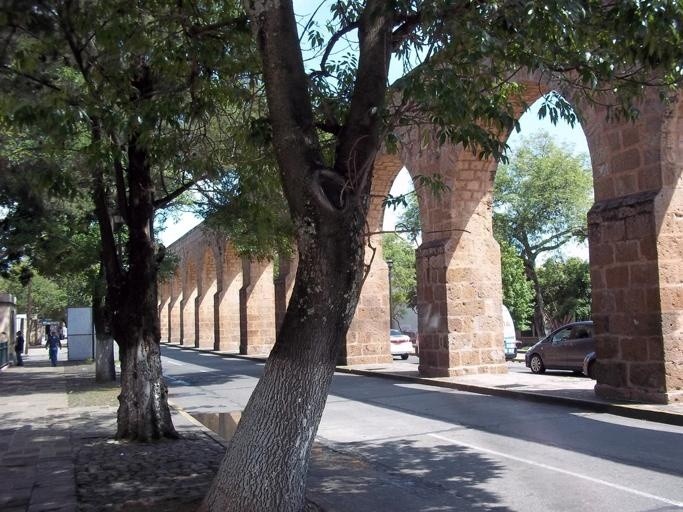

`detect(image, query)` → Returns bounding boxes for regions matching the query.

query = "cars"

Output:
[390,328,415,359]
[525,321,595,376]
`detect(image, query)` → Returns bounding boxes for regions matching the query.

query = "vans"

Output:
[502,304,522,360]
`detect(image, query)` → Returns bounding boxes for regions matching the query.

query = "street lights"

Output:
[386,258,393,329]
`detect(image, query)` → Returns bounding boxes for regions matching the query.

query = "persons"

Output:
[13,331,23,366]
[45,330,61,366]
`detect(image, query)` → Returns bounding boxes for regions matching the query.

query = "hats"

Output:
[51,329,57,333]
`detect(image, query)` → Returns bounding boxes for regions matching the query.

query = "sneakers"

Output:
[16,363,23,366]
[51,364,57,367]
